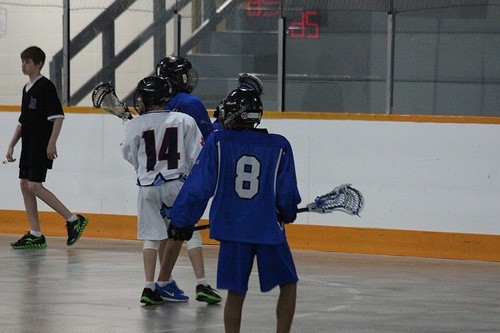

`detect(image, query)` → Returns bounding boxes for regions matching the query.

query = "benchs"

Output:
[179,0,500,117]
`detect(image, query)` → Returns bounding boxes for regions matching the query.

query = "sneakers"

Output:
[195,283,222,304]
[65,215,89,246]
[10,231,49,249]
[170,280,184,294]
[139,287,164,305]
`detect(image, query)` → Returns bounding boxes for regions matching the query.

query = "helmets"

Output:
[132,76,175,114]
[154,56,198,94]
[217,87,263,130]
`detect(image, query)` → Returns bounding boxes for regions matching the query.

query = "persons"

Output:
[120,75,222,305]
[7,46,89,249]
[155,56,212,303]
[167,89,302,333]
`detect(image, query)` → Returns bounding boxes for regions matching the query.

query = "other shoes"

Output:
[154,282,189,302]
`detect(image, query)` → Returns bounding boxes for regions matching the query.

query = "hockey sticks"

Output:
[89,83,134,120]
[192,183,365,230]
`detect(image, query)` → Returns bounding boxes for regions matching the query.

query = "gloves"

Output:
[279,214,297,223]
[167,224,194,241]
